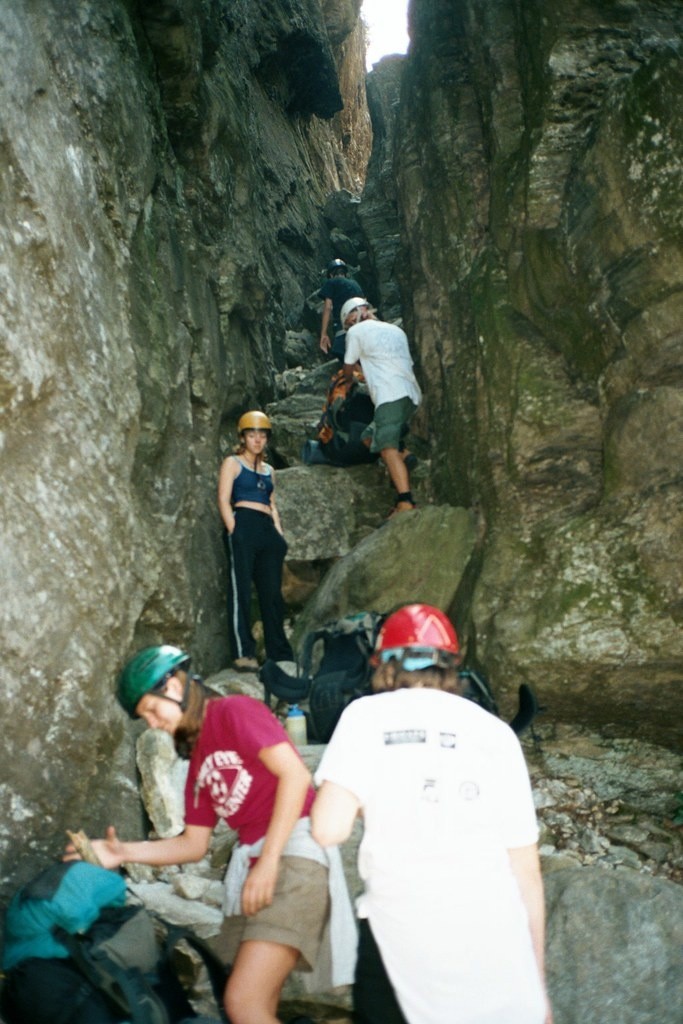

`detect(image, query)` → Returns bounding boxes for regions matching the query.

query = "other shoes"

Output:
[389,449,417,488]
[236,657,260,670]
[386,499,416,521]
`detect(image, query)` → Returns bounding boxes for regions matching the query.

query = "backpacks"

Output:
[317,366,374,466]
[16,906,198,1023]
[259,610,379,748]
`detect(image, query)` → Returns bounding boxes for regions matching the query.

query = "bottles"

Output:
[284,703,309,747]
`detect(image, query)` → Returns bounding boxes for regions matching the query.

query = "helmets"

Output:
[341,297,370,330]
[114,645,190,721]
[372,603,462,669]
[237,411,272,436]
[325,259,347,279]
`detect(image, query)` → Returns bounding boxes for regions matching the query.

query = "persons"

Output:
[340,298,423,518]
[317,258,365,358]
[217,410,293,670]
[309,604,547,1024]
[63,645,358,1024]
